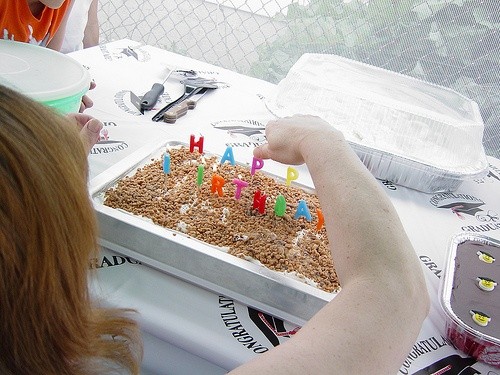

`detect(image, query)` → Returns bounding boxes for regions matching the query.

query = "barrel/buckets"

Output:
[0,39,91,115]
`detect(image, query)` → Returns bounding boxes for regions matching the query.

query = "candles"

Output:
[163,134,326,229]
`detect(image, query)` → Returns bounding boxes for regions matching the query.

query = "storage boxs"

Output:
[263,51,488,193]
[440,231,500,369]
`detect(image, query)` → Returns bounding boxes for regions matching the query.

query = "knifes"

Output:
[165,87,209,124]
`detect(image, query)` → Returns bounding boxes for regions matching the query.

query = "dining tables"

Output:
[64,39,500,375]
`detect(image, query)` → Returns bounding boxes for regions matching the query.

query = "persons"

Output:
[1,85,430,374]
[0,0,75,51]
[60,0,100,55]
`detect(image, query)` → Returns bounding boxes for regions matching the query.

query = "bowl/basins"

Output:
[263,51,490,194]
